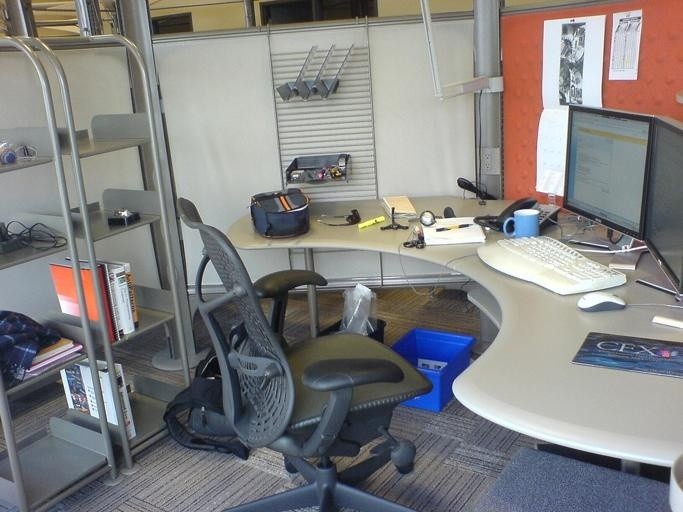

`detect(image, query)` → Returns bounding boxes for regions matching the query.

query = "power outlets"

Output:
[477,146,500,175]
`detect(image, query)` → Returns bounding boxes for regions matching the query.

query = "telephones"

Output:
[497,197,561,233]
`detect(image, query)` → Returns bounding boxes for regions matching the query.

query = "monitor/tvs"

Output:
[562,104,655,269]
[641,116,683,329]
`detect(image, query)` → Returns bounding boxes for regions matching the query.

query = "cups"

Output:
[503,208,539,238]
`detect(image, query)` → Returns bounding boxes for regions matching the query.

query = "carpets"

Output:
[472,448,671,512]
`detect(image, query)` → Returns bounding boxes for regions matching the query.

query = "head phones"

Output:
[0,140,37,168]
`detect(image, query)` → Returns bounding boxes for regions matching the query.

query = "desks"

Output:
[224,194,680,467]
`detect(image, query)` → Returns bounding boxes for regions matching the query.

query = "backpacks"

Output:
[161,319,248,461]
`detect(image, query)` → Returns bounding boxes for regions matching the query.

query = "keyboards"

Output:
[477,234,627,295]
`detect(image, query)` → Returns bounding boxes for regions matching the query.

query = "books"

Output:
[422,215,484,246]
[11,332,82,378]
[50,254,138,340]
[383,195,416,219]
[59,357,137,442]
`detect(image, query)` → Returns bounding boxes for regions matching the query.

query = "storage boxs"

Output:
[391,328,475,413]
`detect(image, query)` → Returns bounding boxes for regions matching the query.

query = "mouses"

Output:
[576,292,625,311]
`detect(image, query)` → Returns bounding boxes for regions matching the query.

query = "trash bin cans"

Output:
[337,318,388,371]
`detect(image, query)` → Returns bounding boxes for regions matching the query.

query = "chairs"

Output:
[173,198,433,512]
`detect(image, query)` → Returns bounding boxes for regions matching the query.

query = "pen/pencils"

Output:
[436,223,473,232]
[357,216,385,229]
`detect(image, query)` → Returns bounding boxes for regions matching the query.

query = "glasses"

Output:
[315,209,361,226]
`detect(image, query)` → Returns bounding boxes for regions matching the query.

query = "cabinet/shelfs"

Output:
[17,33,196,476]
[0,37,121,511]
